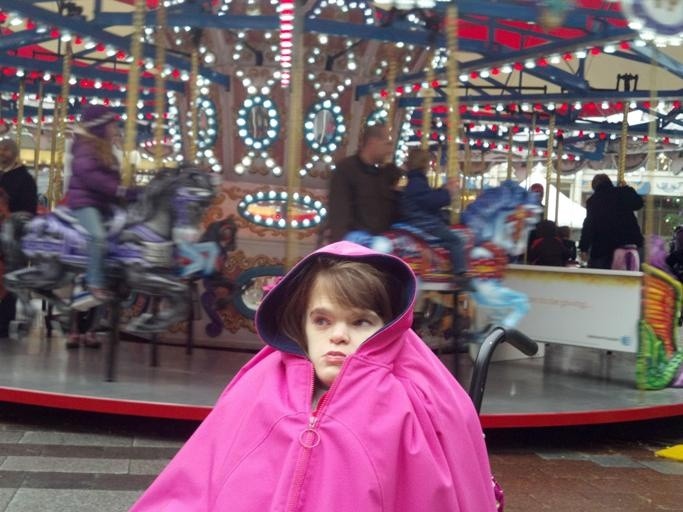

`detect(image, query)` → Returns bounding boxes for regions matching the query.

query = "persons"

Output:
[65,104,145,304]
[392,145,477,295]
[0,137,41,270]
[665,225,682,325]
[578,173,645,268]
[328,121,407,251]
[527,182,577,267]
[122,242,499,511]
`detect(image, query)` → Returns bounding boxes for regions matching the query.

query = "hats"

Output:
[78,103,117,129]
